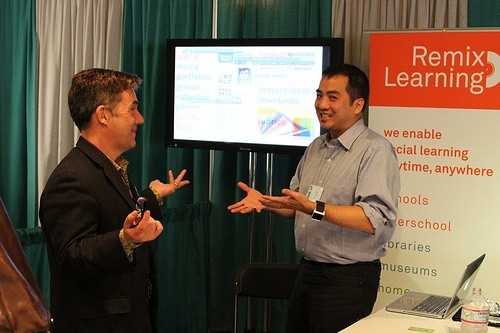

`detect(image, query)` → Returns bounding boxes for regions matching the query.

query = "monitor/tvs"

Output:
[165,38,345,154]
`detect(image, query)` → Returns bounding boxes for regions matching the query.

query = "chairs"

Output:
[234,264,298,333]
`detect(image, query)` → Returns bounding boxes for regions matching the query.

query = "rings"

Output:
[174,180,179,186]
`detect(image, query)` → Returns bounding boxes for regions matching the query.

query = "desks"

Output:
[337,307,500,333]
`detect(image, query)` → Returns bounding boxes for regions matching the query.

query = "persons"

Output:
[227,63,401,332]
[39,67,191,332]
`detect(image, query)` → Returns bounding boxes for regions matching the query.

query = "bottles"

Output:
[459,287,490,333]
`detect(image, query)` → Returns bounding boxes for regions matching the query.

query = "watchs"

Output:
[312,199,327,222]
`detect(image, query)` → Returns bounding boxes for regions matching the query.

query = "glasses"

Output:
[129,197,147,229]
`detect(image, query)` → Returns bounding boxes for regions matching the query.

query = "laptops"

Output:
[387,254,485,320]
